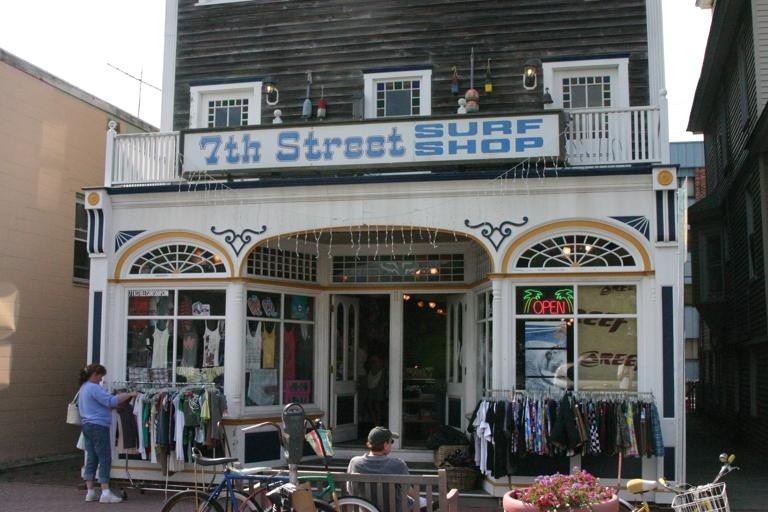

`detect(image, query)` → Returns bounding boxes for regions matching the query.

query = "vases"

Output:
[502,488,620,512]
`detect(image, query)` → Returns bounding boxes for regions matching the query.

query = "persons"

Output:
[345,426,434,512]
[76,363,139,503]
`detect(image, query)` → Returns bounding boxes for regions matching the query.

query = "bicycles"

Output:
[616,452,741,512]
[159,419,380,512]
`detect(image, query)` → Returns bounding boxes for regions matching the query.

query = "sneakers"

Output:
[85,494,99,501]
[99,493,121,503]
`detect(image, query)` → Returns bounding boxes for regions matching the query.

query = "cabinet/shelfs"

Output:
[402,377,445,425]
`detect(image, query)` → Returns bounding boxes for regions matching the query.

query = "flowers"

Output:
[513,465,617,512]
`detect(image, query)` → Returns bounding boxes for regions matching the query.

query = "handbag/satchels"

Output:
[66,404,82,425]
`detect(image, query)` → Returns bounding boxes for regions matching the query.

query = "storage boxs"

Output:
[444,467,480,492]
[433,444,472,468]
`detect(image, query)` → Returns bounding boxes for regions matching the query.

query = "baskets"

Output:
[671,482,731,512]
[434,444,479,493]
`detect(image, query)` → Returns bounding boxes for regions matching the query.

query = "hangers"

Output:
[470,385,655,421]
[96,379,223,405]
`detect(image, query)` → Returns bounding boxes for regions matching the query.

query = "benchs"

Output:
[231,465,459,512]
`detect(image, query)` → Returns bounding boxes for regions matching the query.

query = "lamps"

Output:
[521,58,542,90]
[264,76,279,105]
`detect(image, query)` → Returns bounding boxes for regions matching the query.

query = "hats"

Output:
[368,426,399,444]
